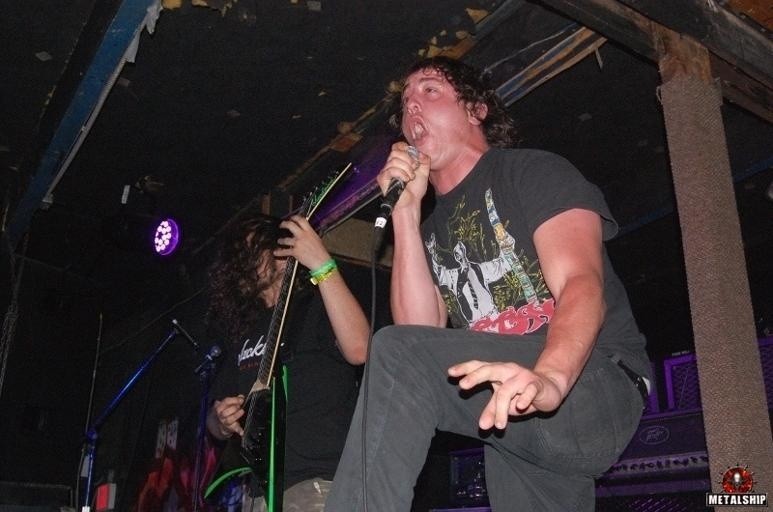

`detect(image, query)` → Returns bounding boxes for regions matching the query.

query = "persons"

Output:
[203,211,374,512]
[322,51,652,511]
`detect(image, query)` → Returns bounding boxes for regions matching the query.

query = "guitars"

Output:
[201,156,358,512]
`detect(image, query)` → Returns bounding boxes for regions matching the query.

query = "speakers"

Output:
[450,407,714,512]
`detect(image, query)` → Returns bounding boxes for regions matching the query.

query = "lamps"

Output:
[107,207,188,258]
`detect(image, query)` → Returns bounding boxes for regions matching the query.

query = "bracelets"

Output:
[309,259,339,286]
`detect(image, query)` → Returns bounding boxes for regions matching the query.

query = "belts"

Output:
[618,360,648,406]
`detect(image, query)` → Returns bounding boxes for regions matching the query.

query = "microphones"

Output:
[373,145,419,252]
[172,320,200,351]
[194,345,221,375]
